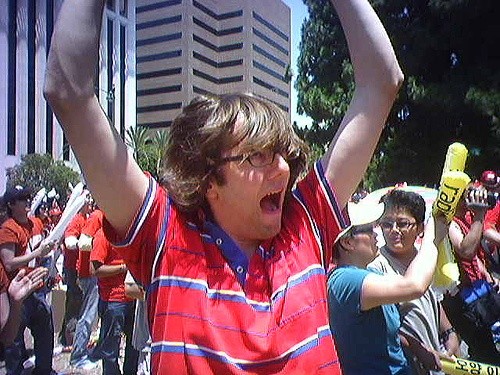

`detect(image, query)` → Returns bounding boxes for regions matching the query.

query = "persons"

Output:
[40,0,405,375]
[0,181,143,375]
[326,168,499,375]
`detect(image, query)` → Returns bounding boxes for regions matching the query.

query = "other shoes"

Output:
[80,360,99,370]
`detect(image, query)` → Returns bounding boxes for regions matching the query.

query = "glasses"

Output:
[379,217,418,230]
[342,225,374,239]
[217,146,302,169]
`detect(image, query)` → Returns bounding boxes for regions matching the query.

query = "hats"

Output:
[333,201,384,248]
[4,184,35,203]
